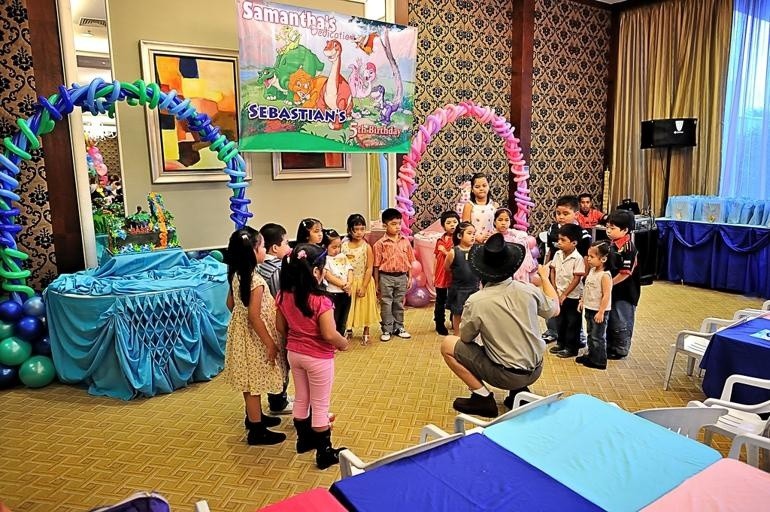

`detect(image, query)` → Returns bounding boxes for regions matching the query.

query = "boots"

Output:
[433,303,448,335]
[247,421,286,445]
[313,428,347,470]
[293,406,317,453]
[245,408,281,427]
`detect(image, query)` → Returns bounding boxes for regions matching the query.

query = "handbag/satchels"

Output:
[618,198,642,214]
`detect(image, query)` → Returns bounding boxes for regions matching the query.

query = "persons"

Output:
[296,217,323,245]
[372,208,416,341]
[258,221,290,299]
[435,231,562,420]
[218,225,286,446]
[339,213,382,346]
[432,192,642,371]
[275,242,353,470]
[319,228,354,343]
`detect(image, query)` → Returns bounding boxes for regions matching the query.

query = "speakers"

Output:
[642,117,697,149]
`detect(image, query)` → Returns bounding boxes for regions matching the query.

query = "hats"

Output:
[468,233,526,282]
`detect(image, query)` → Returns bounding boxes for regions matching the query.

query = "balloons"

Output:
[405,256,431,307]
[2,74,254,297]
[209,250,224,262]
[388,98,534,244]
[0,296,57,391]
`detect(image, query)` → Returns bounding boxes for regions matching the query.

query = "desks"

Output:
[654,217,769,299]
[413,229,537,302]
[42,263,232,400]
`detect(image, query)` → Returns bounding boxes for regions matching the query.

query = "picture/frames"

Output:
[272,151,352,180]
[139,40,253,184]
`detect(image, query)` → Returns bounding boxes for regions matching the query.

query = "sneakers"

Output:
[361,332,369,345]
[345,332,352,344]
[576,350,624,371]
[269,396,295,414]
[453,393,499,418]
[392,328,411,338]
[542,328,587,358]
[504,395,527,409]
[380,331,393,341]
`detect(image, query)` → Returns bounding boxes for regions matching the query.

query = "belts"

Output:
[498,365,538,375]
